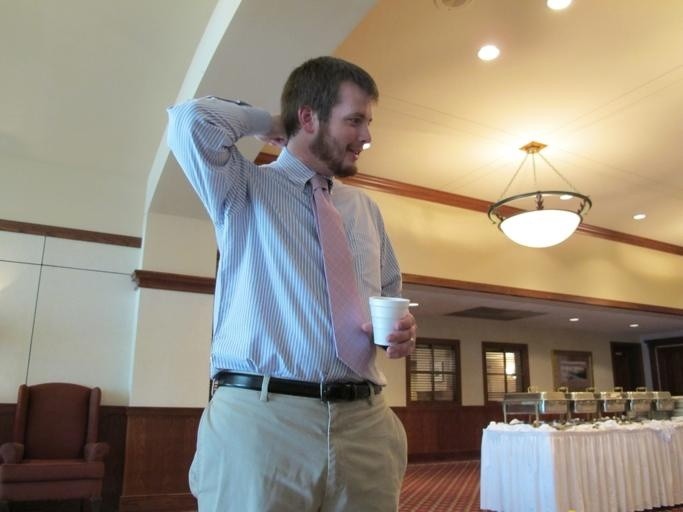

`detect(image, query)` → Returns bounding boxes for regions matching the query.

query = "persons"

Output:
[168,55,417,512]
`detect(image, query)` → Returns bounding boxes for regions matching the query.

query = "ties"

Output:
[310,174,377,379]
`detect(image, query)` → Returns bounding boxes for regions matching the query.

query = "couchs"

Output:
[2,381,111,510]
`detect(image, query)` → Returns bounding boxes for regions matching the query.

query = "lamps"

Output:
[482,141,596,250]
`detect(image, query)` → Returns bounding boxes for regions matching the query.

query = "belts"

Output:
[217,371,383,403]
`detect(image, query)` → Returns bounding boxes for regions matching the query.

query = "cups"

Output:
[369,294,409,346]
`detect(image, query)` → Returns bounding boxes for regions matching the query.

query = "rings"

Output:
[410,336,415,344]
